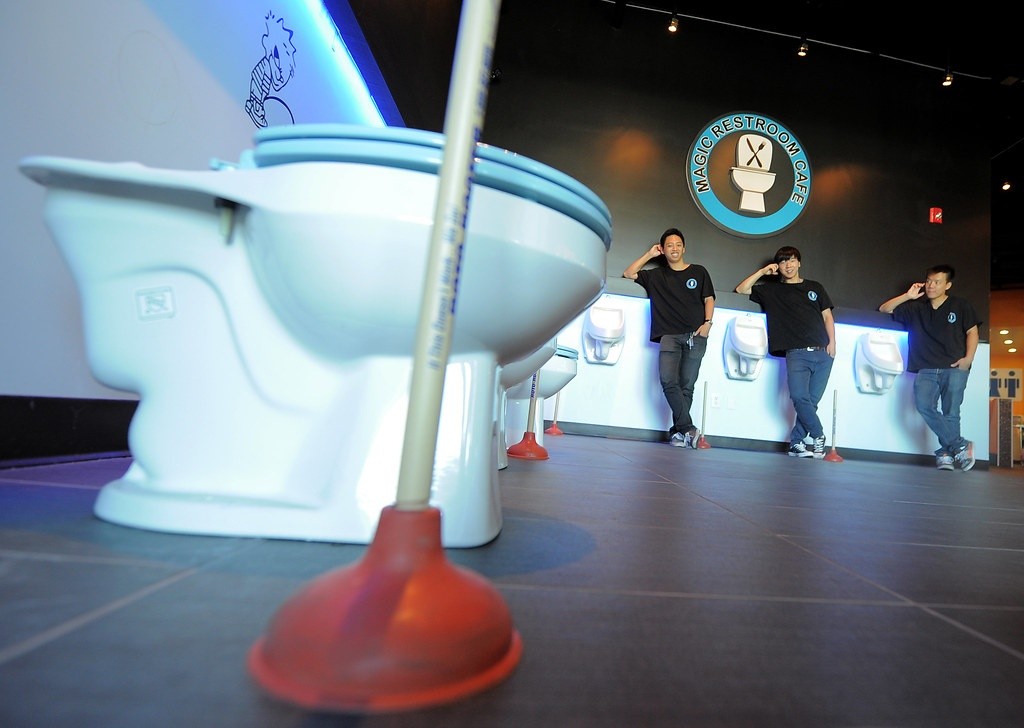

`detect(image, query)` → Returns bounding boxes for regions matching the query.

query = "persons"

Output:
[878,264,983,473]
[624,228,716,450]
[735,247,836,460]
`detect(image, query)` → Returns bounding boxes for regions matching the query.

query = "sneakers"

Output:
[957,441,975,471]
[670,438,686,446]
[813,436,827,458]
[788,445,813,457]
[937,457,954,471]
[685,428,700,449]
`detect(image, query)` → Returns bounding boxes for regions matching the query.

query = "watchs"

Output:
[704,320,713,325]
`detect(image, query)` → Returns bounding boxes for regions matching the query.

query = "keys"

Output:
[686,332,695,349]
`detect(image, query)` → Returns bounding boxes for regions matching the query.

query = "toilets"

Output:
[506,346,578,452]
[489,336,558,469]
[22,123,611,540]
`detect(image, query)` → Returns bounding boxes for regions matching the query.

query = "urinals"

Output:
[854,333,904,393]
[723,315,768,382]
[582,307,625,365]
